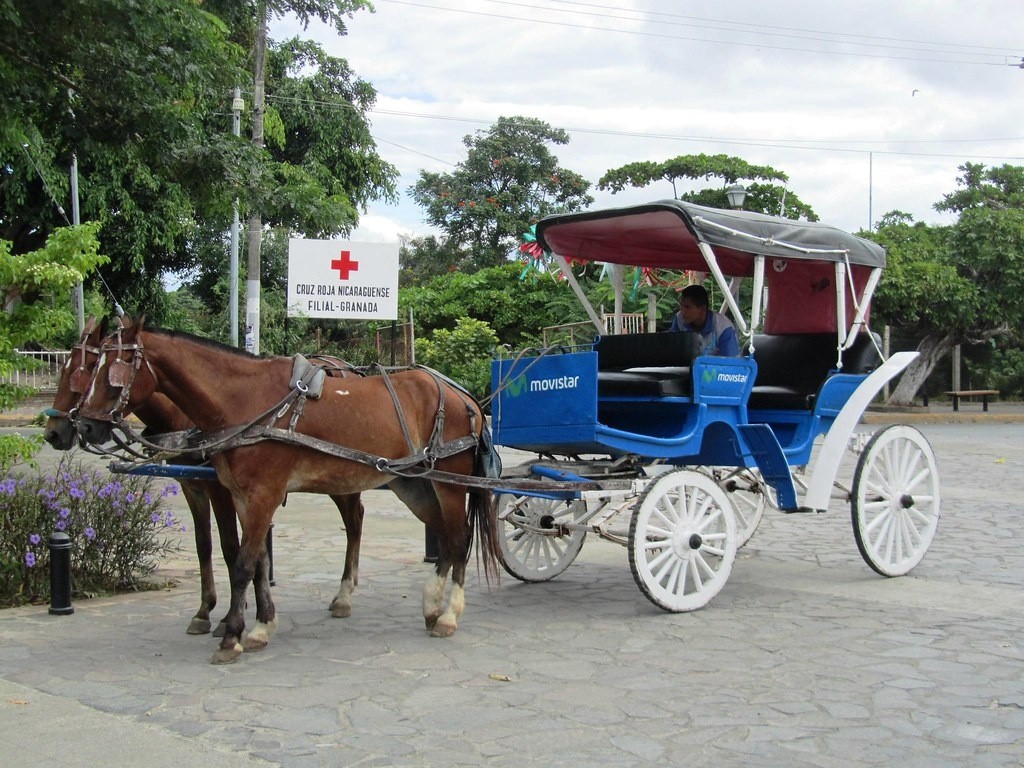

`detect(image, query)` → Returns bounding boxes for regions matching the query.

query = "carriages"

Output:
[42,196,943,668]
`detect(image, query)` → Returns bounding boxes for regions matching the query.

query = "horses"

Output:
[44,313,499,665]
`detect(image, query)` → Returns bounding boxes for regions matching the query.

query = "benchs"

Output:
[740,331,885,409]
[944,389,1000,411]
[593,330,706,397]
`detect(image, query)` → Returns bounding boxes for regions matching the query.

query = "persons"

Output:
[668,285,740,358]
